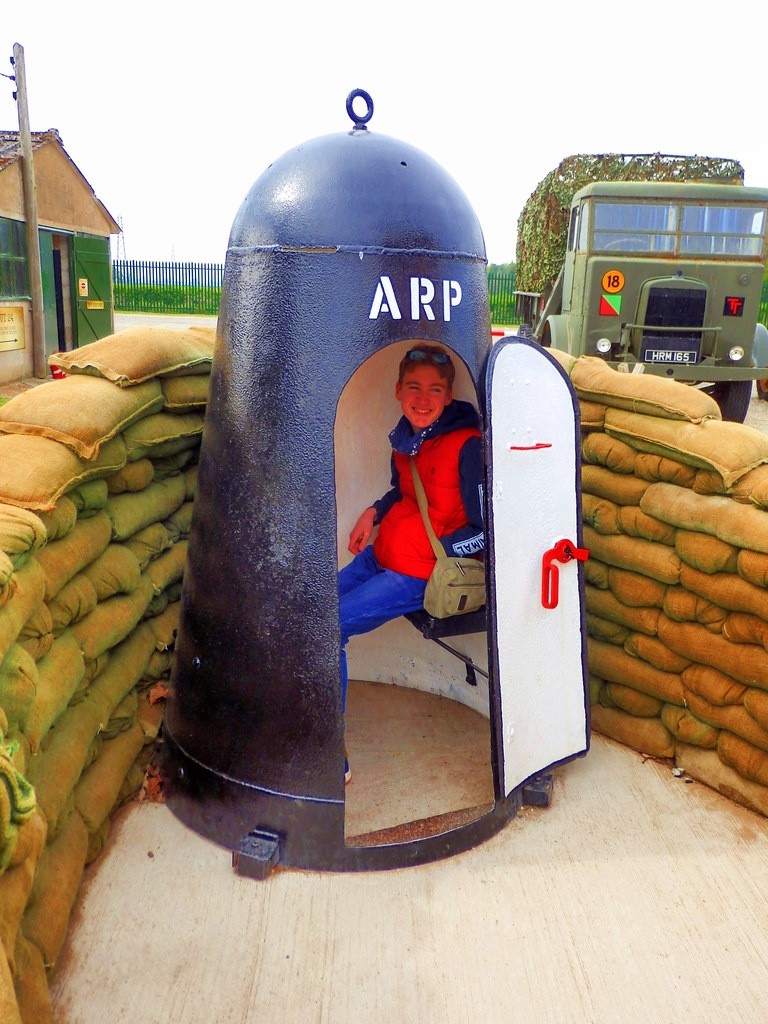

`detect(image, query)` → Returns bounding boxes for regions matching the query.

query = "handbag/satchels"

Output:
[423,557,488,619]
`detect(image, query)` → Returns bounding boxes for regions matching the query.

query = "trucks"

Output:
[511,152,768,425]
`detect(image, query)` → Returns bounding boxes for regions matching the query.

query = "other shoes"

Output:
[344,761,352,784]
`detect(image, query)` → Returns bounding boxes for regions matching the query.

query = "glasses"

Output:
[405,350,450,364]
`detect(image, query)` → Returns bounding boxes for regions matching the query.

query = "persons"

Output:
[336,344,488,786]
